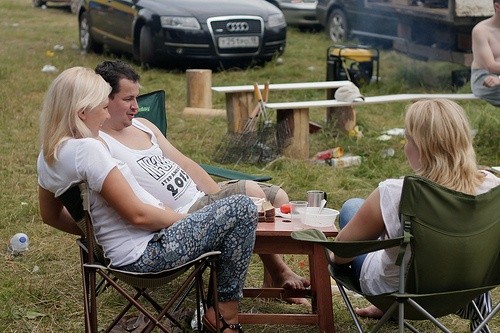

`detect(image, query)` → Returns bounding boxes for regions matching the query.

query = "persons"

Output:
[36,66,258,333]
[470,1,500,109]
[92,61,313,308]
[329,98,500,320]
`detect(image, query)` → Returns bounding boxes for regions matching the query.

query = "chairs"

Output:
[133,89,273,182]
[287,173,500,333]
[55,176,221,333]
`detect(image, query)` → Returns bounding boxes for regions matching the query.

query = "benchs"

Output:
[211,80,356,136]
[262,93,481,159]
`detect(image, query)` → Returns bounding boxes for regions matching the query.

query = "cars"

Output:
[76,0,287,72]
[31,0,80,14]
[315,0,449,45]
[266,0,320,26]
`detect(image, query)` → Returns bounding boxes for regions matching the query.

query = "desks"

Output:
[204,205,340,333]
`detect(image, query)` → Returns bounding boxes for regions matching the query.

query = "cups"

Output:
[289,201,308,230]
[307,190,328,208]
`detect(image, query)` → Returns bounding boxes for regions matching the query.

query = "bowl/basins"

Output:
[304,207,340,228]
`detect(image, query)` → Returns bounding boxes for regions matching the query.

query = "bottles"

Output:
[191,300,205,331]
[313,147,344,159]
[10,232,29,251]
[326,156,362,168]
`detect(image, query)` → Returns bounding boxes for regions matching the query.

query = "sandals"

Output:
[202,302,247,333]
